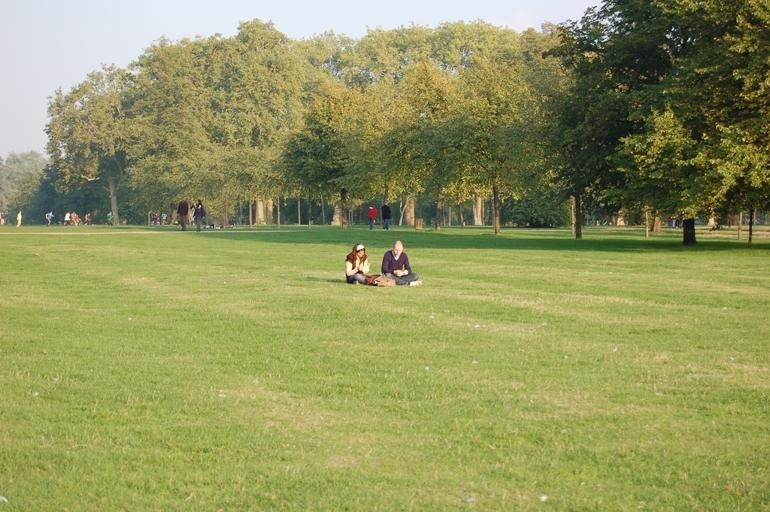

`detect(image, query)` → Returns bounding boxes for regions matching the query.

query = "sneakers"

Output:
[410,280,422,287]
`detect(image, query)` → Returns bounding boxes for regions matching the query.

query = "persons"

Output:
[191,198,205,232]
[151,210,168,226]
[63,209,92,227]
[345,244,382,286]
[177,196,188,231]
[45,211,55,226]
[381,201,391,231]
[16,210,22,228]
[382,241,422,287]
[106,210,113,229]
[1,211,4,224]
[368,204,378,230]
